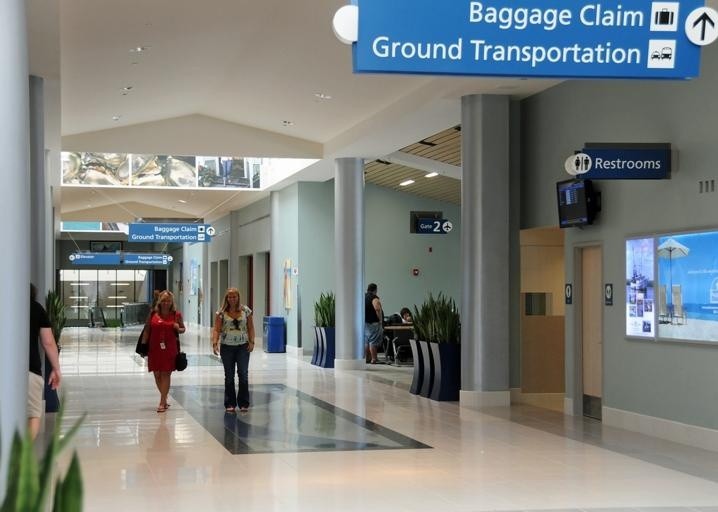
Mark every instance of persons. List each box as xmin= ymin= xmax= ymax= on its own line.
xmin=213 ymin=415 xmax=248 ymax=475
xmin=213 ymin=288 xmax=256 ymax=414
xmin=400 ymin=308 xmax=413 ymax=324
xmin=220 ymin=156 xmax=233 ymax=187
xmin=147 ymin=413 xmax=178 ymax=480
xmin=139 ymin=290 xmax=186 ymax=411
xmin=364 ymin=283 xmax=385 ymax=364
xmin=27 ymin=283 xmax=62 ymax=442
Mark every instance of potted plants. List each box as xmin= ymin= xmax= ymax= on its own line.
xmin=310 ymin=292 xmax=335 ymax=368
xmin=408 ymin=289 xmax=460 ymax=402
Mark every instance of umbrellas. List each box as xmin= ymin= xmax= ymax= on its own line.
xmin=657 ymin=238 xmax=689 ymax=316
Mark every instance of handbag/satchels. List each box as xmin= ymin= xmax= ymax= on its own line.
xmin=176 ymin=348 xmax=190 ymax=373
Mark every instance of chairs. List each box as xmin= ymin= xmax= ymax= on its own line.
xmin=381 ymin=314 xmax=413 ymax=366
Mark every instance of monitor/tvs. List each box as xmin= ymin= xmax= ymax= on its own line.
xmin=556 ymin=178 xmax=599 ymax=228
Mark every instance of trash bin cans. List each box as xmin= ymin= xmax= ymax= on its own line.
xmin=262 ymin=316 xmax=284 ymax=353
